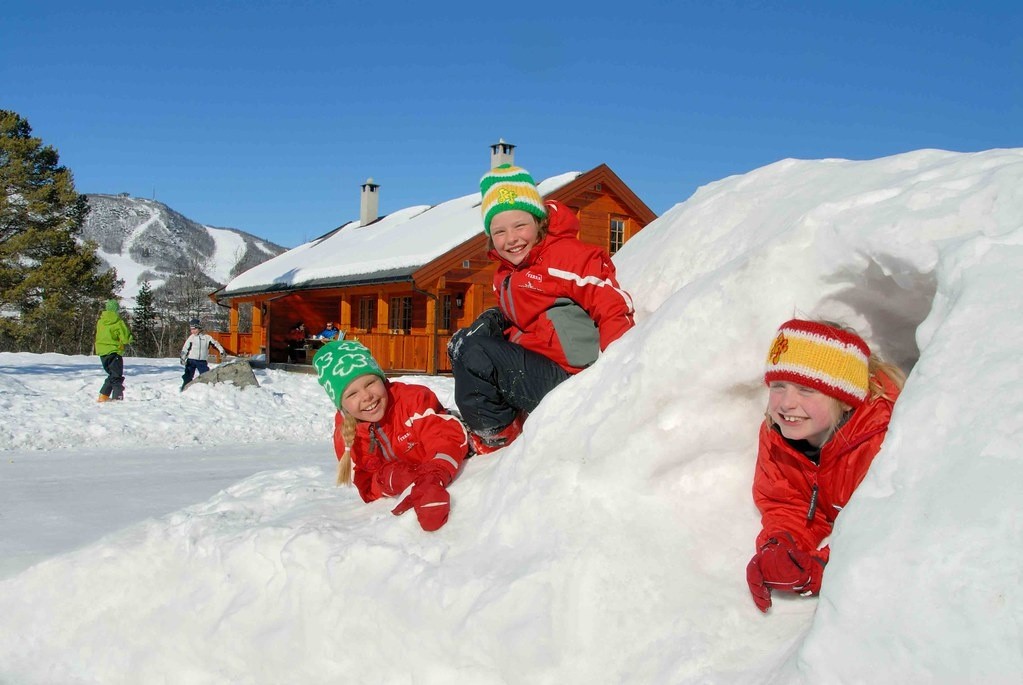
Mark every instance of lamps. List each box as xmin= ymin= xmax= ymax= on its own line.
xmin=456 ymin=293 xmax=463 ymax=308
xmin=262 ymin=304 xmax=267 ymax=315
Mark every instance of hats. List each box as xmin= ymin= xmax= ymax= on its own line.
xmin=190 ymin=319 xmax=204 ymax=328
xmin=312 ymin=339 xmax=388 ymax=411
xmin=479 ymin=163 xmax=548 ymax=238
xmin=106 ymin=299 xmax=119 ymax=312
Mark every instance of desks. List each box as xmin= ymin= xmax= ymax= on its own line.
xmin=306 ymin=339 xmax=330 ymax=345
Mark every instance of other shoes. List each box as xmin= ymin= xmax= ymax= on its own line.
xmin=98 ymin=393 xmax=109 ymax=403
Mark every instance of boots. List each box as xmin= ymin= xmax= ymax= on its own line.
xmin=469 ymin=406 xmax=529 ymax=457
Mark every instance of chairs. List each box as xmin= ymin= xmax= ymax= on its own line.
xmin=288 ymin=345 xmax=310 ymax=363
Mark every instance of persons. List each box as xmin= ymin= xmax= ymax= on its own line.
xmin=179 ymin=319 xmax=225 ymax=391
xmin=745 ymin=319 xmax=908 ymax=612
xmin=316 ymin=322 xmax=339 ymax=339
xmin=95 ymin=300 xmax=133 ymax=403
xmin=447 ymin=163 xmax=635 ymax=456
xmin=312 ymin=341 xmax=469 ymax=532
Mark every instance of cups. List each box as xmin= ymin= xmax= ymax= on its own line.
xmin=313 ymin=335 xmax=316 ymax=340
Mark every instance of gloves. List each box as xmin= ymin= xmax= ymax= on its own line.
xmin=220 ymin=353 xmax=227 ymax=358
xmin=760 ymin=543 xmax=827 ymax=597
xmin=374 ymin=458 xmax=423 ymax=498
xmin=391 ymin=463 xmax=450 ymax=532
xmin=180 ymin=358 xmax=186 ymax=365
xmin=746 ymin=553 xmax=772 ymax=613
